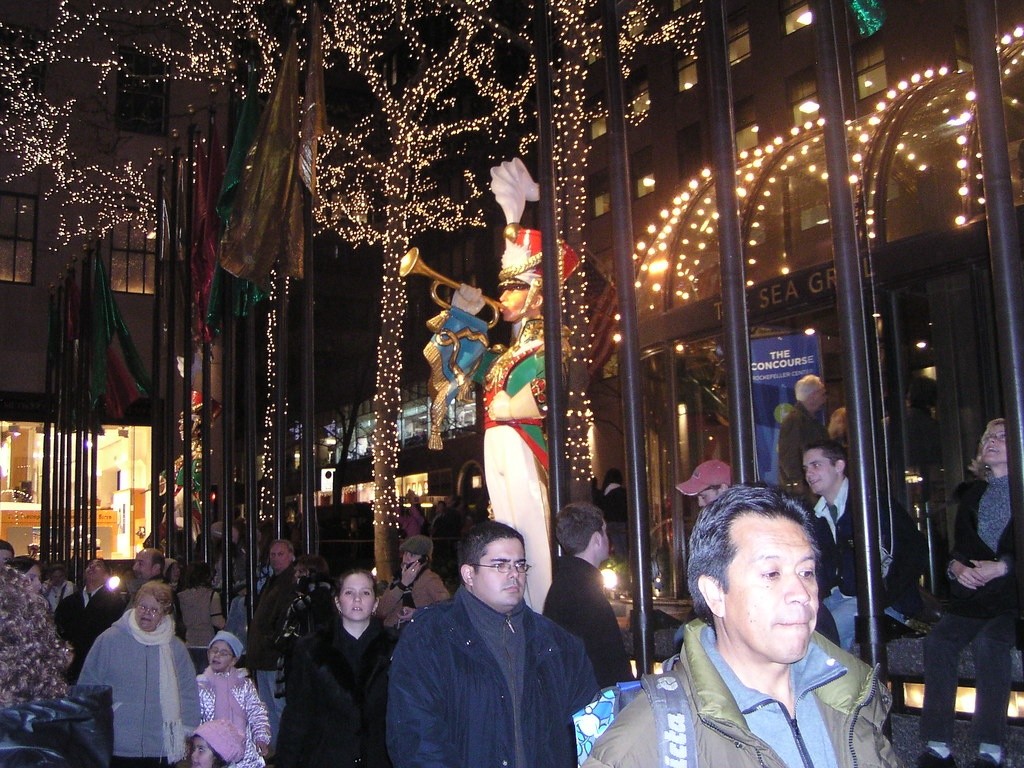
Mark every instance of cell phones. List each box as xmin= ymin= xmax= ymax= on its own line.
xmin=407 ymin=555 xmax=430 ymax=571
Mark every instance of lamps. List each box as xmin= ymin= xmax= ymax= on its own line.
xmin=35 ymin=423 xmax=44 ymax=433
xmin=9 ymin=423 xmax=20 ymax=432
xmin=118 ymin=427 xmax=128 ymax=436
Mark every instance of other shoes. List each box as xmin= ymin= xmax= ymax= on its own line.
xmin=969 ymin=754 xmax=1007 ymax=768
xmin=918 ymin=750 xmax=957 ymax=768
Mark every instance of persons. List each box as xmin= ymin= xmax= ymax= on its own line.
xmin=423 ymin=227 xmax=581 ymax=611
xmin=541 ymin=503 xmax=637 ymax=688
xmin=185 ymin=719 xmax=246 ymax=768
xmin=196 ymin=631 xmax=272 ymax=768
xmin=584 ymin=483 xmax=905 ymax=768
xmin=917 ymin=417 xmax=1024 ymax=768
xmin=0 ymin=563 xmax=114 ymax=768
xmin=76 ymin=581 xmax=202 ymax=768
xmin=386 ymin=520 xmax=599 ymax=768
xmin=801 ymin=442 xmax=923 ymax=652
xmin=0 ymin=517 xmax=343 ymax=750
xmin=777 ymin=374 xmax=945 ymax=527
xmin=379 ymin=534 xmax=450 ymax=629
xmin=274 ymin=568 xmax=401 ymax=768
xmin=158 ymin=392 xmax=223 ymax=537
xmin=589 ymin=467 xmax=628 ymax=563
xmin=675 ymin=459 xmax=731 ymax=507
xmin=320 ymin=493 xmax=473 ymax=586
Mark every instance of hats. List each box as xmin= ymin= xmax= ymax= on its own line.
xmin=193 ymin=719 xmax=246 ymax=763
xmin=675 ymin=459 xmax=731 ymax=495
xmin=400 ymin=535 xmax=433 ymax=559
xmin=209 ymin=630 xmax=244 ymax=659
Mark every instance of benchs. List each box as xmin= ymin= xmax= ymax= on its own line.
xmin=618 ymin=627 xmax=1024 ymax=768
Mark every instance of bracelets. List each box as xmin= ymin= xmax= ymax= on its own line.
xmin=947 ymin=559 xmax=956 ymax=580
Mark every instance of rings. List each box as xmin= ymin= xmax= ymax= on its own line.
xmin=411 ymin=569 xmax=414 ymax=571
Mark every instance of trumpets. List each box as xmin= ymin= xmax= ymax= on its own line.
xmin=398 ymin=248 xmax=504 ymax=330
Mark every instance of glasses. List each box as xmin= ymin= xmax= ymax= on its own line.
xmin=981 ymin=431 xmax=1006 ymax=444
xmin=137 ymin=603 xmax=163 ymax=617
xmin=473 ymin=562 xmax=532 ymax=573
xmin=210 ymin=647 xmax=232 ymax=658
